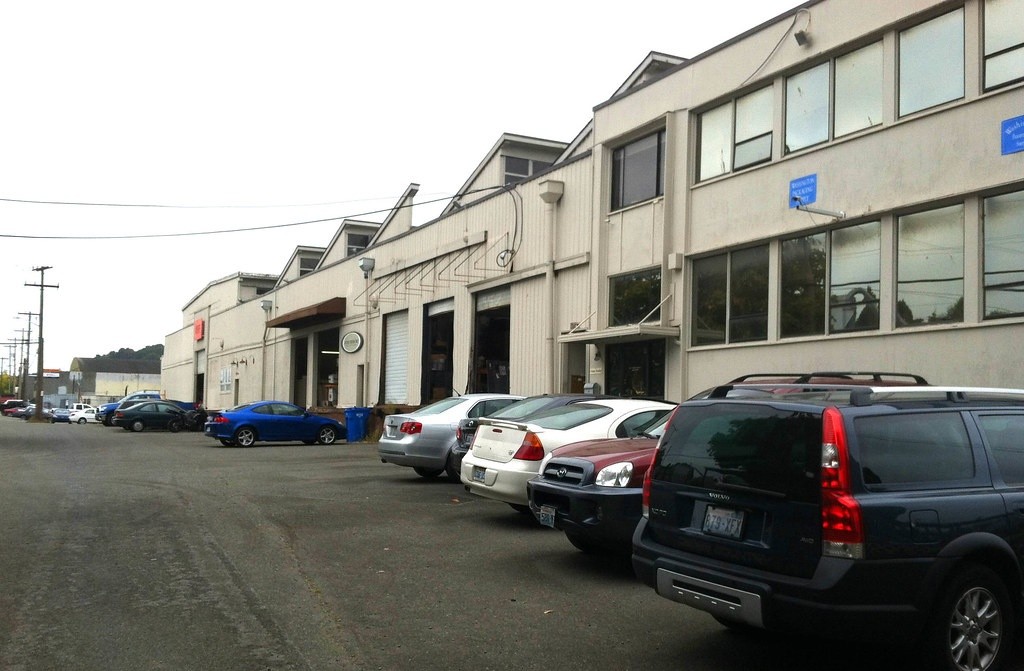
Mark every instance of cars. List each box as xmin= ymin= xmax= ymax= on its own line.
xmin=68 ymin=408 xmax=102 ymax=424
xmin=449 ymin=393 xmax=681 ymax=477
xmin=204 ymin=401 xmax=347 ymax=448
xmin=0 ymin=399 xmax=53 ymax=421
xmin=110 ymin=400 xmax=206 ymax=432
xmin=458 ymin=399 xmax=679 ymax=513
xmin=377 ymin=393 xmax=525 ymax=483
xmin=52 ymin=409 xmax=73 ymax=424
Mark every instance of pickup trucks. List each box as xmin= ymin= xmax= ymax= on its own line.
xmin=51 ymin=403 xmax=93 ymax=413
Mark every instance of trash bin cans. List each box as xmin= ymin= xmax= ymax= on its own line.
xmin=345 ymin=407 xmax=370 ymax=445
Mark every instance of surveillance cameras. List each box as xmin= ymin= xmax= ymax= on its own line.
xmin=793 ymin=195 xmax=802 ymax=201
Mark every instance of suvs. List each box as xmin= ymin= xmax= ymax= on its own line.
xmin=628 ymin=373 xmax=1024 ymax=671
xmin=526 ymin=369 xmax=937 ymax=551
xmin=94 ymin=390 xmax=160 ymax=427
